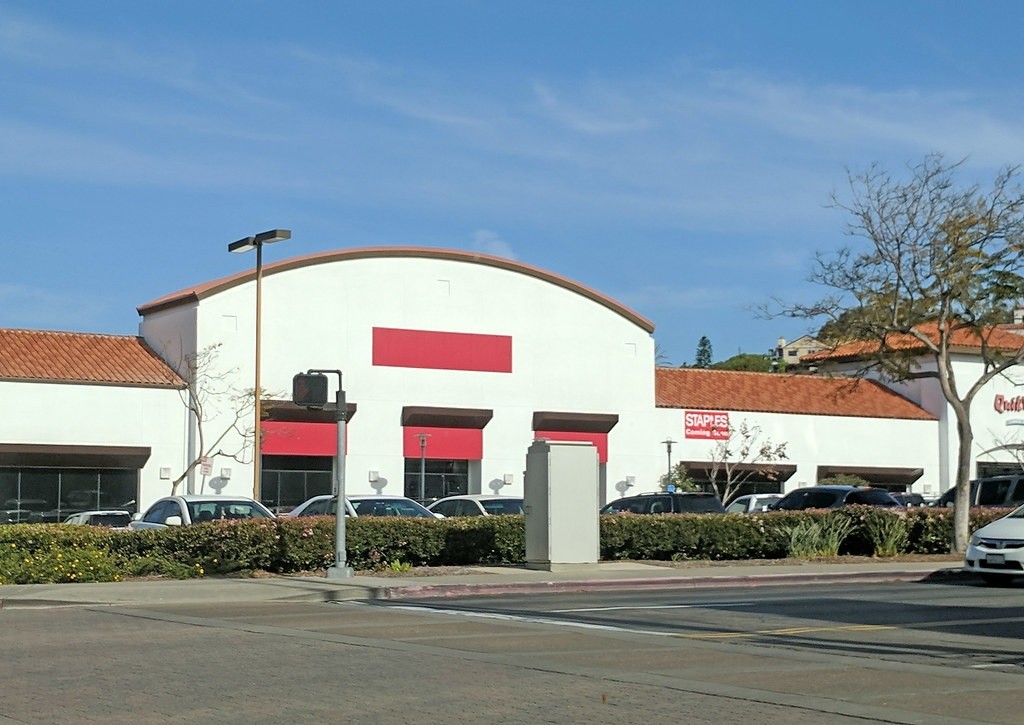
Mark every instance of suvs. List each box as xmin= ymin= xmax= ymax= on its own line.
xmin=600 ymin=491 xmax=729 ymax=514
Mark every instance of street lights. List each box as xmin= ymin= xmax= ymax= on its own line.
xmin=660 ymin=437 xmax=678 ymax=492
xmin=228 ymin=228 xmax=292 ymax=502
xmin=414 ymin=433 xmax=433 ymax=507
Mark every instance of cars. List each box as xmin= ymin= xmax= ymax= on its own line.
xmin=932 ymin=475 xmax=1024 ymax=508
xmin=275 ymin=494 xmax=448 ymax=520
xmin=128 ymin=494 xmax=277 ymax=530
xmin=414 ymin=494 xmax=524 ymax=518
xmin=964 ymin=504 xmax=1024 ymax=583
xmin=889 ymin=492 xmax=927 ymax=508
xmin=62 ymin=510 xmax=133 ymax=532
xmin=767 ymin=486 xmax=902 ymax=511
xmin=725 ymin=493 xmax=786 ymax=514
